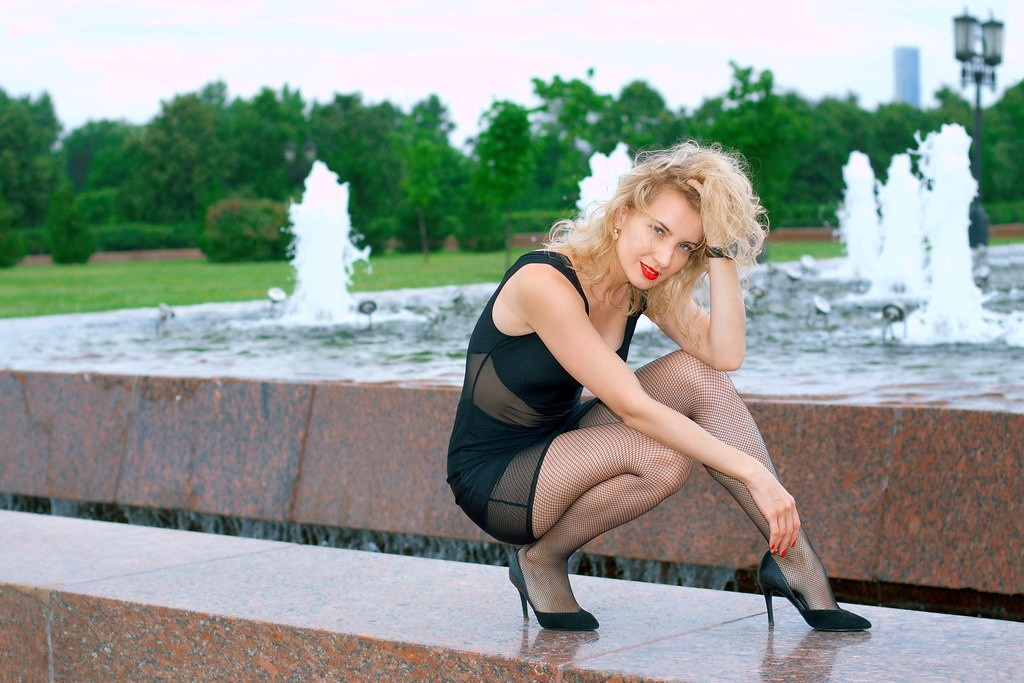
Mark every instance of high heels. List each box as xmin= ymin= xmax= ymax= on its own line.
xmin=757 ymin=549 xmax=872 ymax=631
xmin=509 ymin=545 xmax=600 ymax=631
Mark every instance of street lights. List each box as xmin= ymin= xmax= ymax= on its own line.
xmin=954 ymin=3 xmax=1003 ymax=249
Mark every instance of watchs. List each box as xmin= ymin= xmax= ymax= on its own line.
xmin=702 ymin=243 xmax=739 ymax=260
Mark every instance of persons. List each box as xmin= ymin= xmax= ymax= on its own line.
xmin=445 ymin=136 xmax=872 ymax=631
